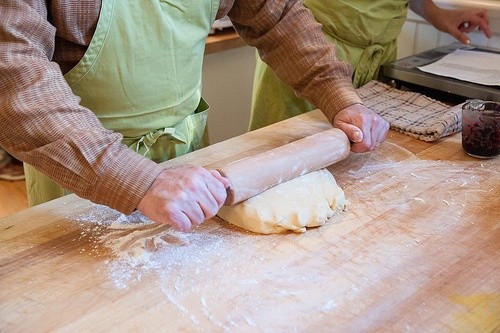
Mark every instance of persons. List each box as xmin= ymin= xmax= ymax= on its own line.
xmin=0 ymin=0 xmax=390 ymax=231
xmin=248 ymin=0 xmax=493 ymax=132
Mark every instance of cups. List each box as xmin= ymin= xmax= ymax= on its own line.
xmin=460 ymin=100 xmax=500 ymax=159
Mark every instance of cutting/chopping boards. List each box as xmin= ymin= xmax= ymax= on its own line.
xmin=0 ymin=107 xmax=500 ymax=333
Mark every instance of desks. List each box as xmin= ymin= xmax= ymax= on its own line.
xmin=202 ymin=33 xmax=257 ymax=145
xmin=0 ymin=108 xmax=500 ymax=333
